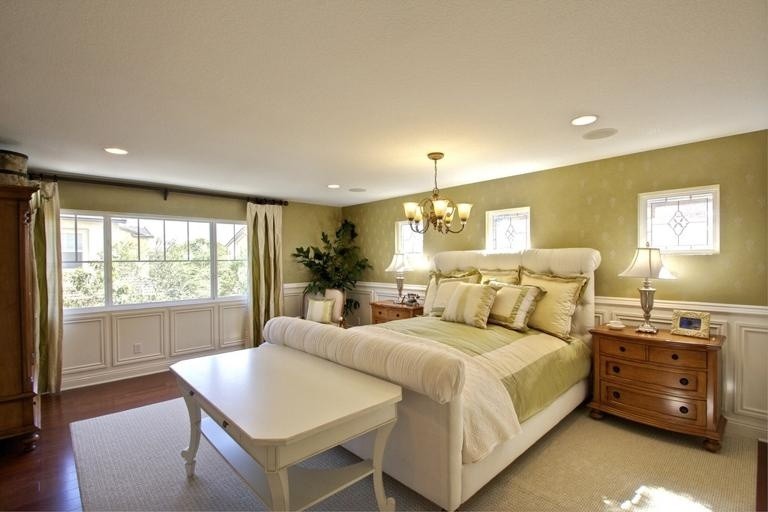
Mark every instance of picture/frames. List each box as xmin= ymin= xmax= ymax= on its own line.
xmin=671 ymin=310 xmax=711 ymax=339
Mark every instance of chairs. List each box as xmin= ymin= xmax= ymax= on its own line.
xmin=295 ymin=286 xmax=347 ymax=329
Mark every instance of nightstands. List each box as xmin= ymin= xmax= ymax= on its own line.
xmin=369 ymin=300 xmax=423 ymax=324
xmin=589 ymin=323 xmax=727 ymax=451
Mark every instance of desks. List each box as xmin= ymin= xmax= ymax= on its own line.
xmin=169 ymin=344 xmax=402 ymax=512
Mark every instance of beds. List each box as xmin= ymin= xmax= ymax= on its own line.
xmin=262 ymin=248 xmax=602 ymax=512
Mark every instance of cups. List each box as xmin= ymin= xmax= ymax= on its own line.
xmin=610 ymin=321 xmax=622 ymax=326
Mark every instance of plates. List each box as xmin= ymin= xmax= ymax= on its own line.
xmin=606 ymin=323 xmax=626 ymax=330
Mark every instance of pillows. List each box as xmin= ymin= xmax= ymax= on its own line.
xmin=423 ymin=265 xmax=589 ymax=343
xmin=306 ymin=297 xmax=335 ymax=325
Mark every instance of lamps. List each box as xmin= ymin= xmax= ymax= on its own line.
xmin=617 ymin=241 xmax=677 ymax=334
xmin=384 ymin=253 xmax=414 ymax=304
xmin=403 ymin=152 xmax=474 ymax=235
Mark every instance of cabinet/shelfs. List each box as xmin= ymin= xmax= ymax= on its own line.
xmin=0 ymin=184 xmax=42 ymax=452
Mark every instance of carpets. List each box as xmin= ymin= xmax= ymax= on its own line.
xmin=68 ymin=397 xmax=768 ymax=512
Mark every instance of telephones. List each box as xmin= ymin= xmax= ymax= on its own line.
xmin=401 ymin=293 xmax=420 ymax=306
